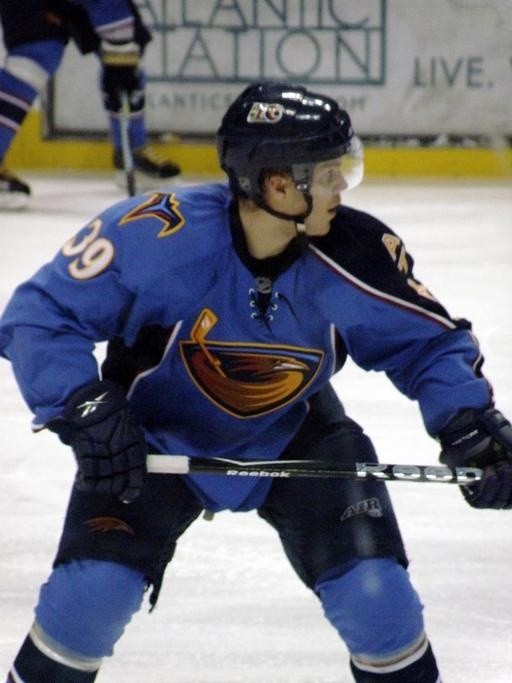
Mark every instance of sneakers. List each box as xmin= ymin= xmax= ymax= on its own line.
xmin=112 ymin=143 xmax=181 ymax=178
xmin=0 ymin=171 xmax=30 ymax=195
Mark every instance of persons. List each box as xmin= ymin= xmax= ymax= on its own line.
xmin=1 ymin=84 xmax=510 ymax=681
xmin=1 ymin=0 xmax=181 ymax=195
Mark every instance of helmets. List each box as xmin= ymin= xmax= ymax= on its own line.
xmin=214 ymin=79 xmax=365 ymax=201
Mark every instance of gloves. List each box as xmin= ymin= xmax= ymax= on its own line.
xmin=437 ymin=405 xmax=511 ymax=510
xmin=45 ymin=382 xmax=147 ymax=506
